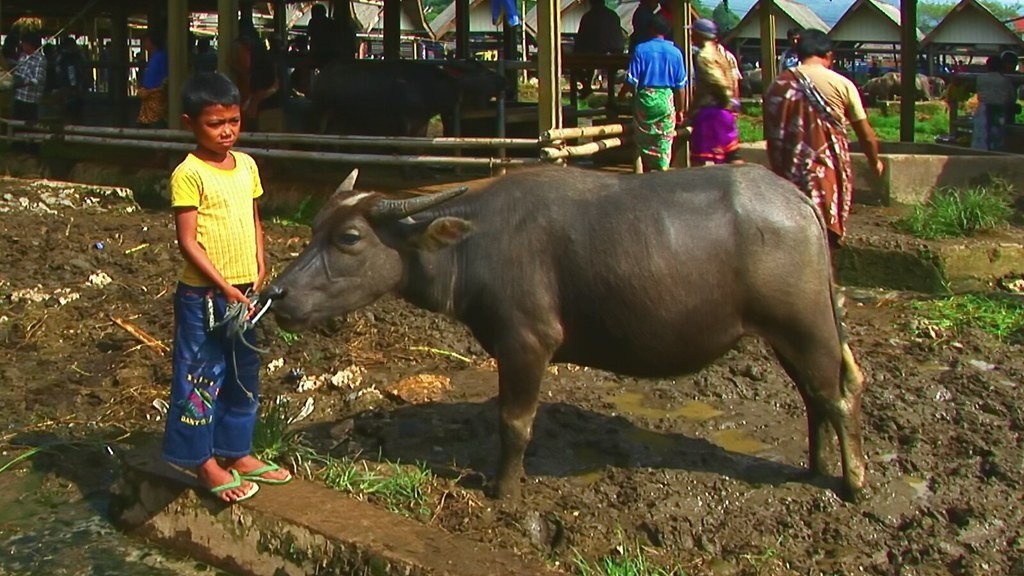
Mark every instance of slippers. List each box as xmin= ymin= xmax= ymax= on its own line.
xmin=240 ymin=459 xmax=292 ymax=484
xmin=209 ymin=468 xmax=260 ymax=503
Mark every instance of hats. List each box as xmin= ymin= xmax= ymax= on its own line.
xmin=690 ymin=18 xmax=718 ymax=39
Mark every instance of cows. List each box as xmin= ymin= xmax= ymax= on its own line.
xmin=259 ymin=164 xmax=868 ymax=499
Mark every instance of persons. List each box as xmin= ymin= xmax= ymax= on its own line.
xmin=2 ymin=26 xmax=113 ymax=161
xmin=866 ymin=62 xmax=880 ymax=82
xmin=574 ymin=0 xmax=674 ymax=115
xmin=944 ymin=60 xmax=968 ymax=78
xmin=161 ymin=71 xmax=292 ymax=503
xmin=779 ymin=28 xmax=803 ymax=71
xmin=618 ymin=14 xmax=687 ymax=174
xmin=976 ymin=51 xmax=1024 ymax=126
xmin=761 ymin=29 xmax=883 ymax=289
xmin=690 ymin=19 xmax=743 ymax=168
xmin=129 ymin=0 xmax=355 ymax=181
